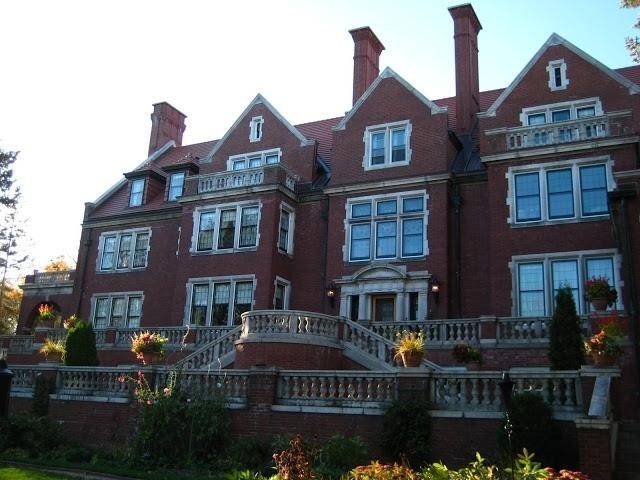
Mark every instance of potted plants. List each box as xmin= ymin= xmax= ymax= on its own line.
xmin=453 ymin=343 xmax=486 ymax=371
xmin=40 ymin=338 xmax=66 ymax=362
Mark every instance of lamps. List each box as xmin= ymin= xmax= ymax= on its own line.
xmin=326 ymin=284 xmax=336 ymax=305
xmin=431 ymin=274 xmax=440 ymax=304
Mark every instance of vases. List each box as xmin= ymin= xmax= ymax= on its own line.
xmin=592 ymin=349 xmax=616 ymax=366
xmin=592 ymin=298 xmax=609 ymax=310
xmin=43 ymin=318 xmax=54 ymax=328
xmin=139 ymin=352 xmax=153 ymax=363
xmin=401 ymin=351 xmax=422 ymax=368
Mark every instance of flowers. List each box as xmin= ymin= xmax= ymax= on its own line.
xmin=130 ymin=331 xmax=168 ymax=358
xmin=393 ymin=329 xmax=431 ymax=360
xmin=583 ymin=310 xmax=629 ymax=357
xmin=38 ymin=303 xmax=58 ymax=319
xmin=582 ymin=276 xmax=619 ymax=308
